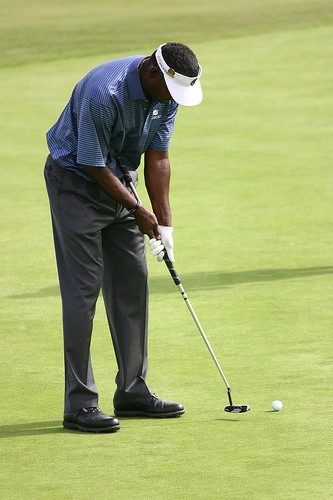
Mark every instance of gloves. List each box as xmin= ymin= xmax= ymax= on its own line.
xmin=148 ymin=224 xmax=175 ymax=264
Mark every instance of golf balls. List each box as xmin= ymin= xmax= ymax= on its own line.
xmin=271 ymin=400 xmax=283 ymax=411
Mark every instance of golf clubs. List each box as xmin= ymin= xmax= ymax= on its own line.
xmin=119 ymin=158 xmax=251 ymax=414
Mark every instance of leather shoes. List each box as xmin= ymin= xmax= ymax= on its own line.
xmin=113 ymin=392 xmax=185 ymax=417
xmin=64 ymin=407 xmax=120 ymax=432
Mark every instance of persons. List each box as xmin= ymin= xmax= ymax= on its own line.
xmin=43 ymin=42 xmax=200 ymax=433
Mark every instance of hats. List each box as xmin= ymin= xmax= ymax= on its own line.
xmin=155 ymin=43 xmax=203 ymax=106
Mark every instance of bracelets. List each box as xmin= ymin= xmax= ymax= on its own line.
xmin=129 ymin=202 xmax=140 ymax=212
